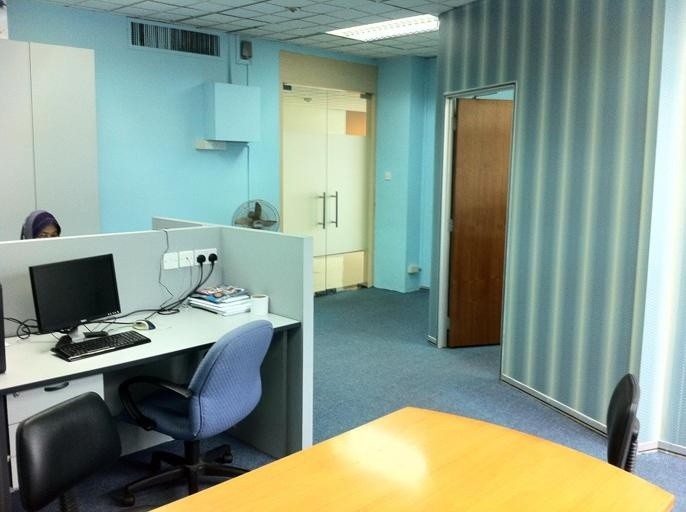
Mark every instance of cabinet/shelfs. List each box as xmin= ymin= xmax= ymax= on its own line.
xmin=0 ymin=38 xmax=100 ymax=245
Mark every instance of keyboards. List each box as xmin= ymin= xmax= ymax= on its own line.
xmin=51 ymin=328 xmax=151 ymax=363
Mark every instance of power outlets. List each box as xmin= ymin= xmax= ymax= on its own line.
xmin=195 ymin=248 xmax=218 ymax=266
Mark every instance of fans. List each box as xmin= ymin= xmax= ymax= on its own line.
xmin=231 ymin=199 xmax=280 ymax=232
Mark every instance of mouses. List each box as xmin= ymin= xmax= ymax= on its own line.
xmin=134 ymin=317 xmax=155 ymax=331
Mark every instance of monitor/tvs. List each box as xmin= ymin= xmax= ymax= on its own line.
xmin=28 ymin=253 xmax=122 ymax=346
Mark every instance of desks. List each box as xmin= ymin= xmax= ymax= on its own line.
xmin=148 ymin=407 xmax=675 ymax=512
xmin=0 ymin=304 xmax=301 ymax=512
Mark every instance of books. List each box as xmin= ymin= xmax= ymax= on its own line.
xmin=186 ymin=282 xmax=253 ymax=317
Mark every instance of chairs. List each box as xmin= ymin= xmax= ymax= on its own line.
xmin=16 ymin=392 xmax=121 ymax=512
xmin=606 ymin=373 xmax=640 ymax=475
xmin=109 ymin=319 xmax=275 ymax=507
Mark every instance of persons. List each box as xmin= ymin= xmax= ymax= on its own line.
xmin=19 ymin=209 xmax=62 ymax=240
